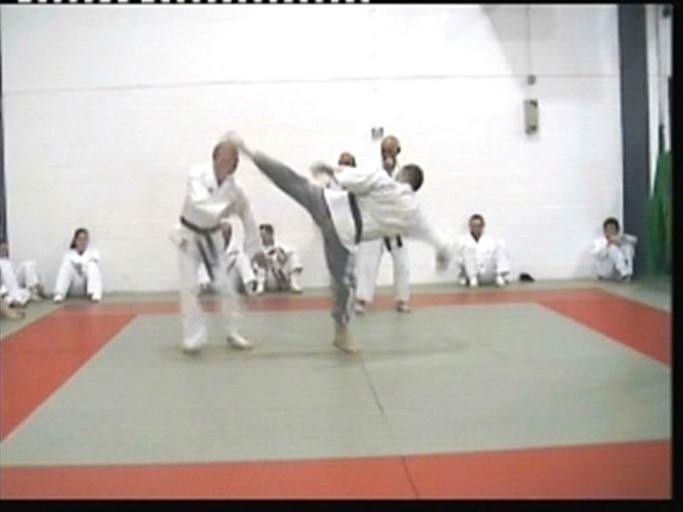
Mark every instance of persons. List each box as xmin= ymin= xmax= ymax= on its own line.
xmin=0 ymin=240 xmax=42 ymax=306
xmin=173 ymin=139 xmax=269 ymax=353
xmin=593 ymin=217 xmax=638 ymax=284
xmin=52 ymin=228 xmax=103 ymax=303
xmin=456 ymin=214 xmax=510 ymax=287
xmin=0 ymin=296 xmax=26 ymax=320
